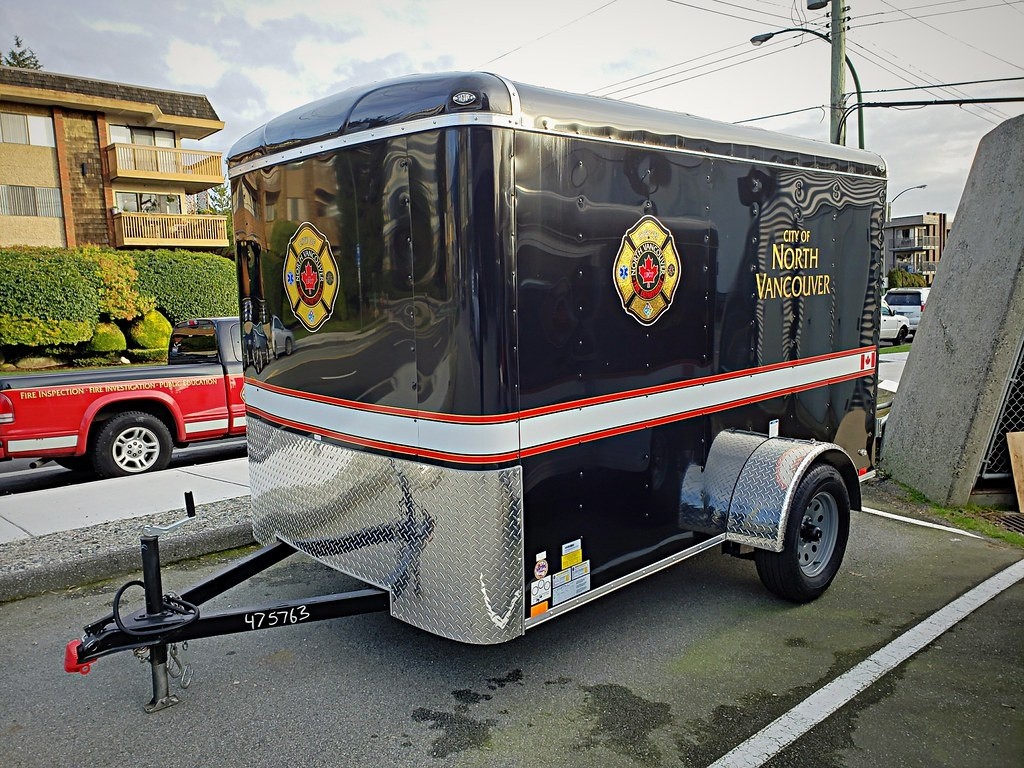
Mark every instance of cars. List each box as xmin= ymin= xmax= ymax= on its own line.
xmin=247 ymin=321 xmax=273 ymax=376
xmin=880 ymin=298 xmax=911 ymax=345
xmin=881 ymin=286 xmax=933 ymax=337
xmin=270 ymin=314 xmax=296 ymax=361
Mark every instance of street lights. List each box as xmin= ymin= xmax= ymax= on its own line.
xmin=887 ymin=182 xmax=929 ymax=221
xmin=749 ymin=27 xmax=865 ymax=152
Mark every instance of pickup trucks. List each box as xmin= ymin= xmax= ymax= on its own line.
xmin=0 ymin=316 xmax=249 ymax=482
